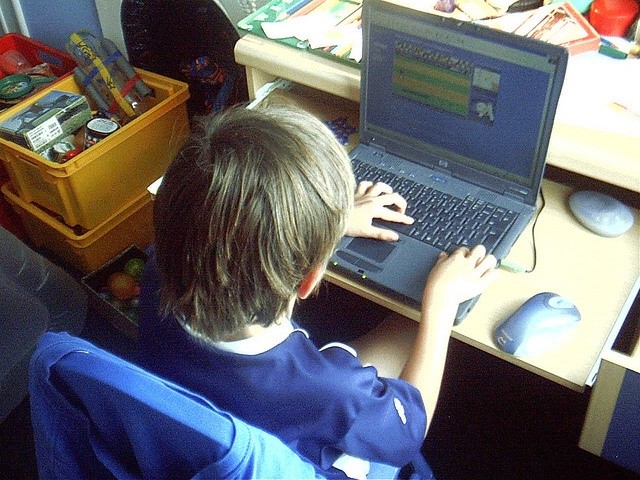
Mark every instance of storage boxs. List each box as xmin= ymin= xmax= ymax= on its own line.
xmin=82 ymin=244 xmax=148 ymax=330
xmin=1 ymin=66 xmax=190 ymax=230
xmin=0 ymin=31 xmax=79 ymax=110
xmin=0 ymin=181 xmax=169 ymax=272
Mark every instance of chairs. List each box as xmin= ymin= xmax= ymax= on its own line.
xmin=28 ymin=329 xmax=326 ymax=480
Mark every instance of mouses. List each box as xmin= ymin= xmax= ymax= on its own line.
xmin=493 ymin=291 xmax=584 ymax=356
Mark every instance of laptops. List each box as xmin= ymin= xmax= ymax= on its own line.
xmin=312 ymin=0 xmax=569 ymax=325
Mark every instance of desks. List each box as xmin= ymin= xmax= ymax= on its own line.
xmin=145 ymin=0 xmax=640 ymax=477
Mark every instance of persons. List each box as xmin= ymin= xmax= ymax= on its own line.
xmin=131 ymin=95 xmax=498 ymax=480
